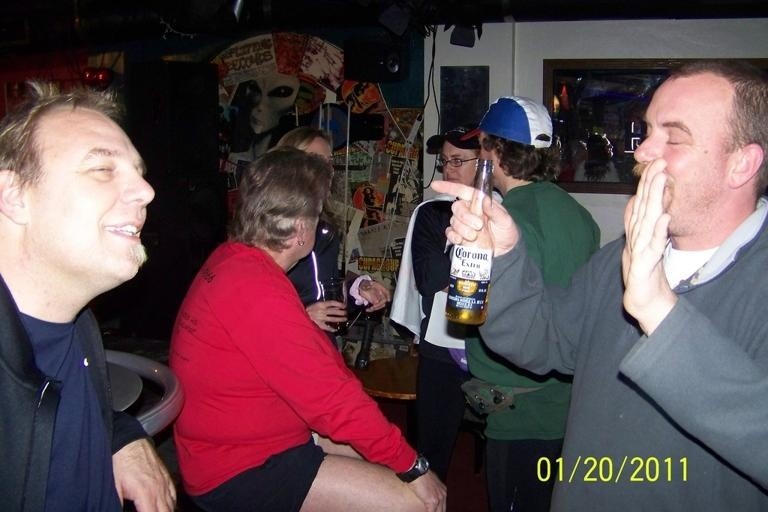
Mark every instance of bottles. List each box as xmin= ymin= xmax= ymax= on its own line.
xmin=444 ymin=158 xmax=494 ymax=326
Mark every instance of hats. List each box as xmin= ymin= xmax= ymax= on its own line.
xmin=460 ymin=96 xmax=554 ymax=149
xmin=425 ymin=124 xmax=483 ymax=155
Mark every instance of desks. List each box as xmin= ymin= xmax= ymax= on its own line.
xmin=347 ymin=355 xmax=418 ymax=401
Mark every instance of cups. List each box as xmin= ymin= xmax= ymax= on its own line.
xmin=323 ymin=277 xmax=348 ymax=335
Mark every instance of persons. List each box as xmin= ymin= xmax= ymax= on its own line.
xmin=460 ymin=94 xmax=602 ymax=512
xmin=428 ymin=58 xmax=768 ymax=512
xmin=389 ymin=124 xmax=505 ymax=482
xmin=272 ymin=126 xmax=391 ymax=354
xmin=169 ymin=147 xmax=448 ymax=511
xmin=0 ymin=76 xmax=178 ymax=511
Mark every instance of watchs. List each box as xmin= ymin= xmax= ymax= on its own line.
xmin=396 ymin=450 xmax=430 ymax=482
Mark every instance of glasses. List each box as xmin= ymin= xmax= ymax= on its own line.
xmin=434 ymin=156 xmax=479 ymax=168
